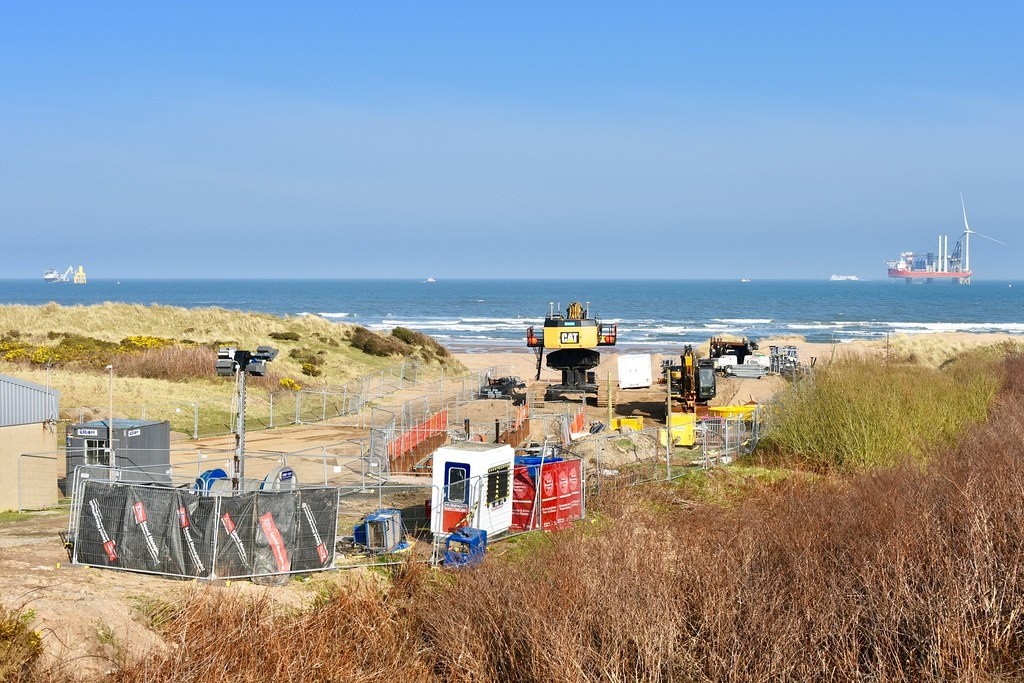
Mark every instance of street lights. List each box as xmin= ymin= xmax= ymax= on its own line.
xmin=105 ymin=363 xmax=114 ymax=483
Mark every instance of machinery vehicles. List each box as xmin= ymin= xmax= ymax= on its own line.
xmin=656 ymin=344 xmax=717 ymax=421
xmin=525 ymin=302 xmax=620 ymax=409
